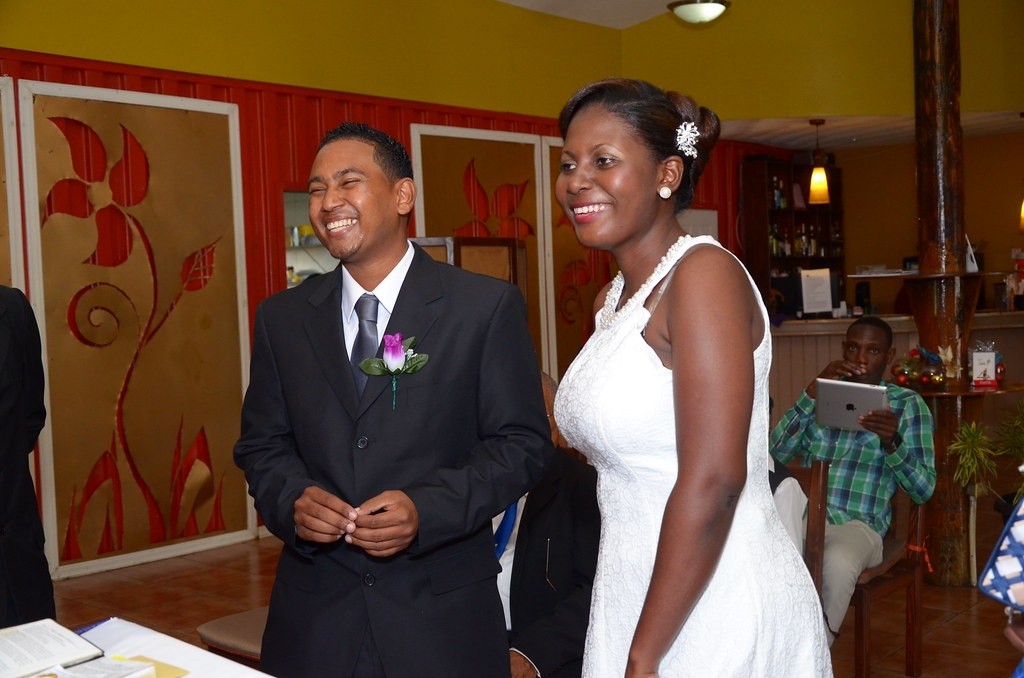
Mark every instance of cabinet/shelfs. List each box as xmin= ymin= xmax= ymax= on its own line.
xmin=847 ymin=265 xmax=1019 ymax=588
xmin=744 ymin=156 xmax=845 ymax=307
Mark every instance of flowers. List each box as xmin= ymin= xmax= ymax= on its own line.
xmin=360 ymin=332 xmax=426 ymax=408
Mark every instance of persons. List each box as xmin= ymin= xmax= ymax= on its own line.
xmin=0 ymin=285 xmax=55 ymax=629
xmin=490 ymin=446 xmax=601 ymax=678
xmin=552 ymin=77 xmax=834 ymax=678
xmin=768 ymin=318 xmax=937 ymax=649
xmin=232 ymin=120 xmax=551 ymax=678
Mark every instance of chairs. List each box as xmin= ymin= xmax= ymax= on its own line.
xmin=854 ymin=487 xmax=934 ymax=678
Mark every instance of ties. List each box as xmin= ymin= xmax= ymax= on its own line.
xmin=492 ymin=501 xmax=517 ymax=560
xmin=350 ymin=293 xmax=380 ymax=402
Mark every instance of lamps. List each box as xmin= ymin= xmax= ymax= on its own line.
xmin=667 ymin=0 xmax=730 ymax=24
xmin=809 ymin=118 xmax=830 ymax=204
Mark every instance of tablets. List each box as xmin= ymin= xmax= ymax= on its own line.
xmin=816 ymin=379 xmax=887 ymax=433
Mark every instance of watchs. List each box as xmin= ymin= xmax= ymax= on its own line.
xmin=879 ymin=431 xmax=903 ymax=452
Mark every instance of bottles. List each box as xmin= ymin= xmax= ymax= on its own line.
xmin=768 ymin=223 xmax=828 ymax=256
xmin=772 ymin=175 xmax=785 ymax=208
